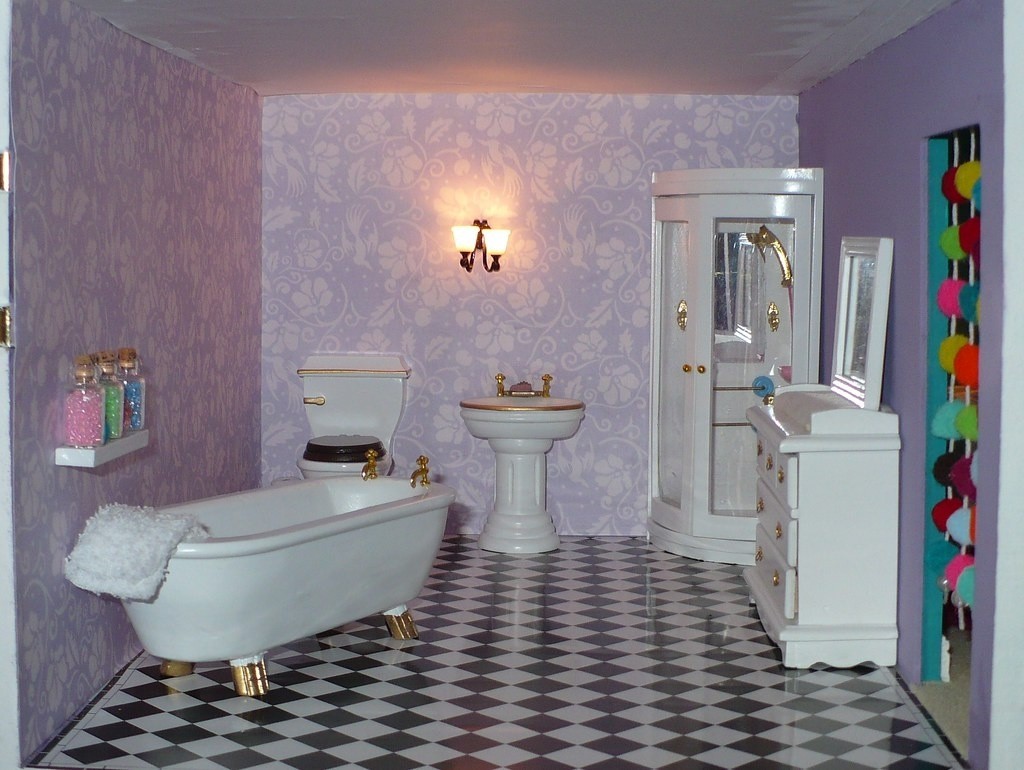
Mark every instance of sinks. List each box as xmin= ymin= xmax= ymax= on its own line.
xmin=459 ymin=388 xmax=588 ymax=442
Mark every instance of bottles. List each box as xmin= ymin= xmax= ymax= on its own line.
xmin=97 ymin=351 xmax=124 ymax=438
xmin=65 ymin=356 xmax=105 ymax=448
xmin=118 ymin=349 xmax=146 ymax=431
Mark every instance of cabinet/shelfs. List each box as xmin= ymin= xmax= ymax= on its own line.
xmin=742 ymin=384 xmax=899 ymax=670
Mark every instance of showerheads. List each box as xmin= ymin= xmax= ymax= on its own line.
xmin=744 ymin=223 xmax=793 ymax=289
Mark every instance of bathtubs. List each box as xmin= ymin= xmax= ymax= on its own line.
xmin=100 ymin=475 xmax=460 ymax=696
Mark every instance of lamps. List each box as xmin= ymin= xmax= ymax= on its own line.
xmin=451 ymin=220 xmax=511 ymax=273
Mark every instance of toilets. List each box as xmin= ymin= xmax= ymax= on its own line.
xmin=297 ymin=348 xmax=413 ymax=480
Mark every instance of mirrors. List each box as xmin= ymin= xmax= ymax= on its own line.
xmin=829 ymin=236 xmax=895 ymax=410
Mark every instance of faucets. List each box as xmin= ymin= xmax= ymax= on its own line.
xmin=360 ymin=447 xmax=379 ymax=482
xmin=407 ymin=454 xmax=433 ymax=488
xmin=495 ymin=372 xmax=554 ymax=398
